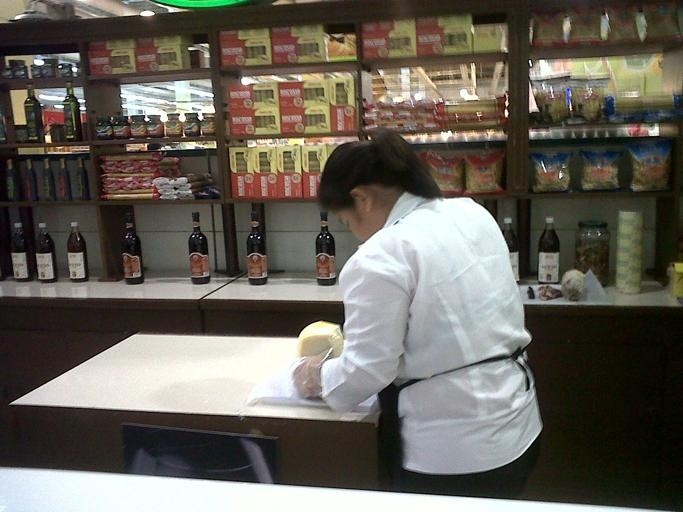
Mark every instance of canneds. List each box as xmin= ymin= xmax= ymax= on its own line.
xmin=95 ymin=111 xmax=216 ymax=140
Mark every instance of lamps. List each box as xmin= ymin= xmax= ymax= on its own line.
xmin=139 ymin=2 xmax=155 ymax=17
xmin=32 ymin=53 xmax=46 ymax=66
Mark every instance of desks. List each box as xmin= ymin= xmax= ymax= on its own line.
xmin=5 ymin=332 xmax=381 ymax=484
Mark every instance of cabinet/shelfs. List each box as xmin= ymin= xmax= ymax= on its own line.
xmin=523 ymin=303 xmax=683 ymax=461
xmin=0 ymin=1 xmax=682 ymax=275
xmin=1 ymin=294 xmax=201 ymax=377
xmin=200 ymin=298 xmax=344 ymax=337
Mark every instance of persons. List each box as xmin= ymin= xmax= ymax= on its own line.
xmin=291 ymin=128 xmax=545 ymax=499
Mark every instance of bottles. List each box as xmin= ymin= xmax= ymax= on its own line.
xmin=0 ymin=102 xmax=7 ymax=143
xmin=121 ymin=212 xmax=144 ymax=284
xmin=23 ymin=159 xmax=38 ymax=201
xmin=246 ymin=211 xmax=269 ymax=285
xmin=503 ymin=217 xmax=520 ymax=286
xmin=59 ymin=158 xmax=72 ymax=201
xmin=315 ymin=210 xmax=337 ymax=285
xmin=64 ymin=82 xmax=83 ymax=140
xmin=537 ymin=217 xmax=561 ymax=284
xmin=24 ymin=82 xmax=44 ymax=141
xmin=4 ymin=158 xmax=20 ymax=201
xmin=10 ymin=221 xmax=34 ymax=281
xmin=42 ymin=158 xmax=56 ymax=201
xmin=77 ymin=157 xmax=90 ymax=200
xmin=67 ymin=222 xmax=89 ymax=282
xmin=188 ymin=211 xmax=211 ymax=284
xmin=35 ymin=222 xmax=58 ymax=282
